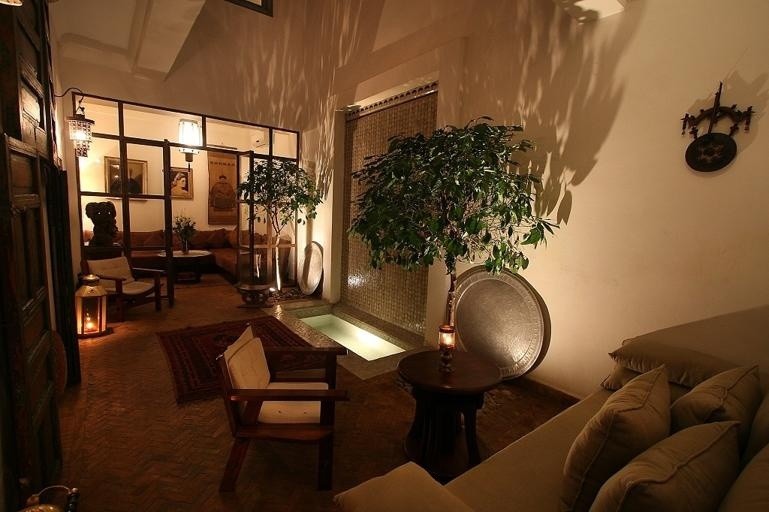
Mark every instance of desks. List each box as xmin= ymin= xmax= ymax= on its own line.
xmin=397 ymin=348 xmax=485 ymax=470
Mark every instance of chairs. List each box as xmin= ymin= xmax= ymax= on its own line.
xmin=214 ymin=326 xmax=338 ymax=495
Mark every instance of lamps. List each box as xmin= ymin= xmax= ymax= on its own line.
xmin=176 ymin=116 xmax=203 ymax=177
xmin=53 ymin=85 xmax=94 ymax=160
xmin=268 ymin=288 xmax=287 ymax=312
xmin=436 ymin=322 xmax=456 ymax=376
xmin=75 ymin=272 xmax=113 ymax=336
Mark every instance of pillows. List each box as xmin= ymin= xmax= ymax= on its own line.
xmin=590 ymin=420 xmax=741 ymax=512
xmin=560 ymin=365 xmax=671 ymax=512
xmin=669 ymin=362 xmax=761 ymax=427
xmin=746 ymin=393 xmax=769 ymax=458
xmin=609 ymin=304 xmax=769 ymax=387
xmin=600 ymin=368 xmax=642 ymax=392
xmin=718 ymin=435 xmax=765 ymax=512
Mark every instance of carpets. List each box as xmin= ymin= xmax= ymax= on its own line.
xmin=154 ymin=317 xmax=341 ymax=407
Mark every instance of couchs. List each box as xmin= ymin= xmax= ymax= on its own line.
xmin=332 ymin=303 xmax=768 ymax=510
